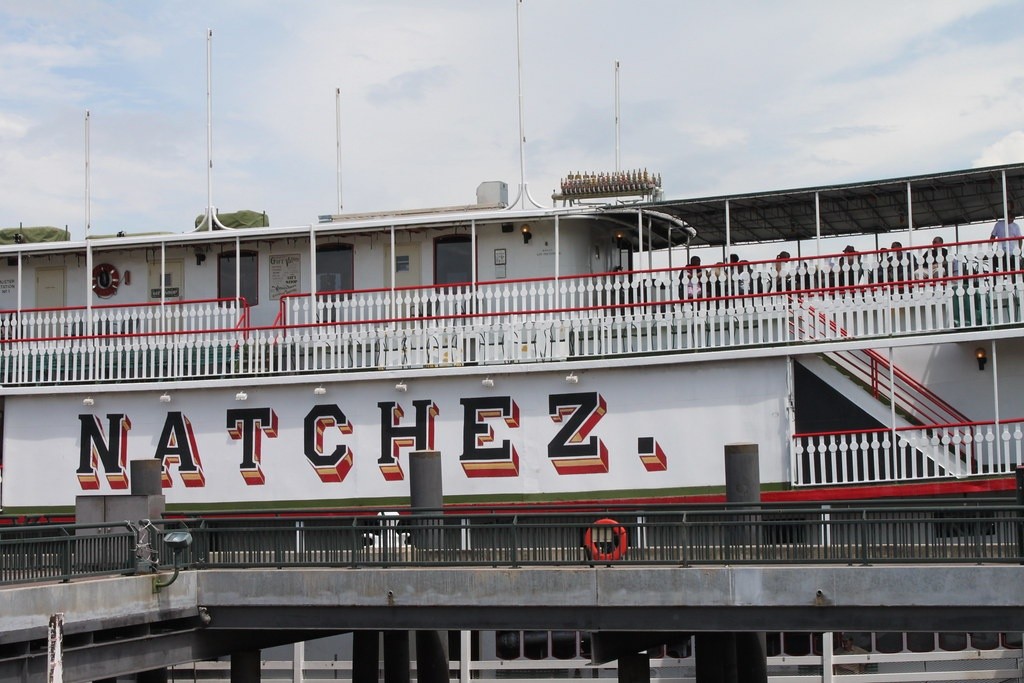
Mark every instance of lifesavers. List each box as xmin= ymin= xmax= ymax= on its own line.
xmin=585 ymin=518 xmax=627 ymax=562
xmin=92 ymin=263 xmax=119 ymax=296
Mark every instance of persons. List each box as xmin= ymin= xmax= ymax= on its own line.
xmin=678 ymin=234 xmax=950 ymax=311
xmin=986 ymin=210 xmax=1023 ymax=288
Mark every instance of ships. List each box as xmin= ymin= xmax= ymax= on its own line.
xmin=0 ymin=3 xmax=1024 ymax=683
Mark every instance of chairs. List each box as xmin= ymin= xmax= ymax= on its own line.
xmin=0 ymin=315 xmax=140 ymax=350
xmin=409 ymin=296 xmax=440 ymax=331
xmin=451 ymin=295 xmax=480 ymax=328
xmin=313 ymin=302 xmax=339 ymax=341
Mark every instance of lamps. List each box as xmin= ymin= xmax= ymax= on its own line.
xmin=151 ymin=531 xmax=194 ymax=594
xmin=520 ymin=223 xmax=532 ymax=244
xmin=193 ymin=246 xmax=207 ymax=266
xmin=974 ymin=346 xmax=989 ymax=371
xmin=615 ymin=229 xmax=626 ymax=249
